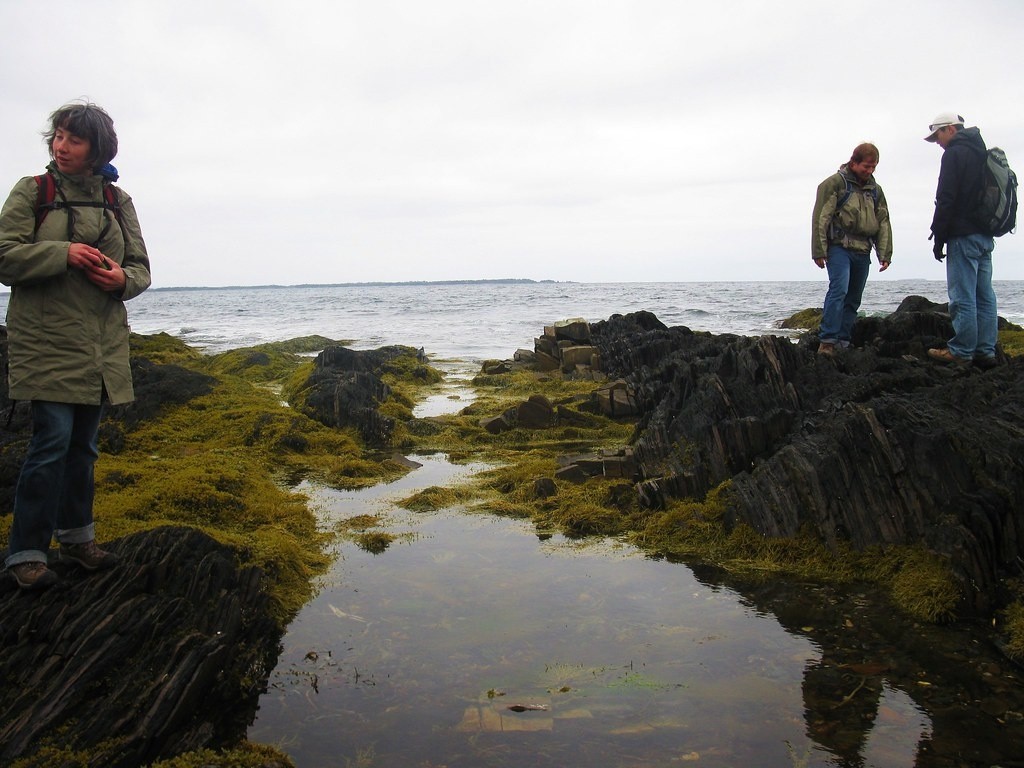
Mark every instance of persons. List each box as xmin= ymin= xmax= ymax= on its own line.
xmin=924 ymin=113 xmax=997 ymax=361
xmin=811 ymin=143 xmax=893 ymax=355
xmin=1 ymin=106 xmax=151 ymax=592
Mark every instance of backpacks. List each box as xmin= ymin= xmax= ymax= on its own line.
xmin=951 ymin=139 xmax=1018 ymax=237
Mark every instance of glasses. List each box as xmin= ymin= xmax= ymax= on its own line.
xmin=929 ymin=123 xmax=952 ymax=132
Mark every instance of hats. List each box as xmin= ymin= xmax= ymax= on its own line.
xmin=924 ymin=112 xmax=965 ymax=142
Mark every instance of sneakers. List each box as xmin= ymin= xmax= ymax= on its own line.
xmin=977 ymin=354 xmax=997 ymax=368
xmin=58 ymin=538 xmax=111 ymax=569
xmin=11 ymin=561 xmax=50 ymax=587
xmin=817 ymin=342 xmax=834 ymax=357
xmin=928 ymin=348 xmax=973 ymax=368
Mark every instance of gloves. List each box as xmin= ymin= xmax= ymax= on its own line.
xmin=933 ymin=238 xmax=946 ymax=262
